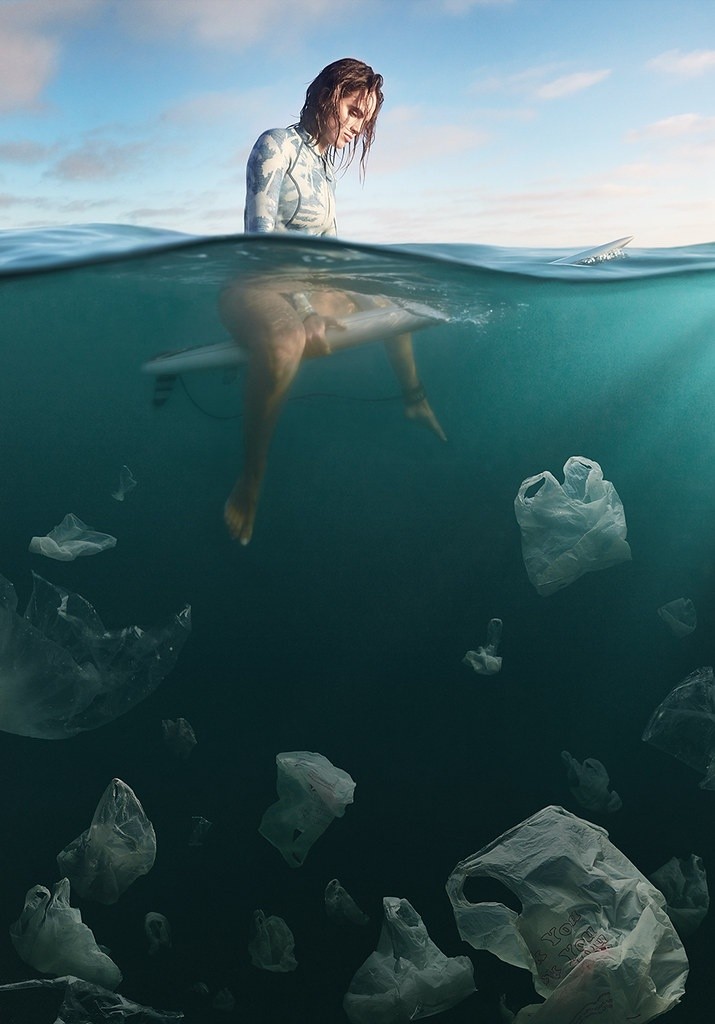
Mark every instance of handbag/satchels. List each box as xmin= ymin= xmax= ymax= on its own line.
xmin=55 ymin=778 xmax=156 ymax=905
xmin=517 ymin=456 xmax=631 ymax=598
xmin=447 ymin=804 xmax=690 ymax=1024
xmin=257 ymin=750 xmax=356 ymax=869
xmin=8 ymin=876 xmax=122 ymax=991
xmin=342 ymin=896 xmax=478 ymax=1024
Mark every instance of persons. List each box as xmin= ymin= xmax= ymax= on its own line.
xmin=244 ymin=58 xmax=383 ymax=235
